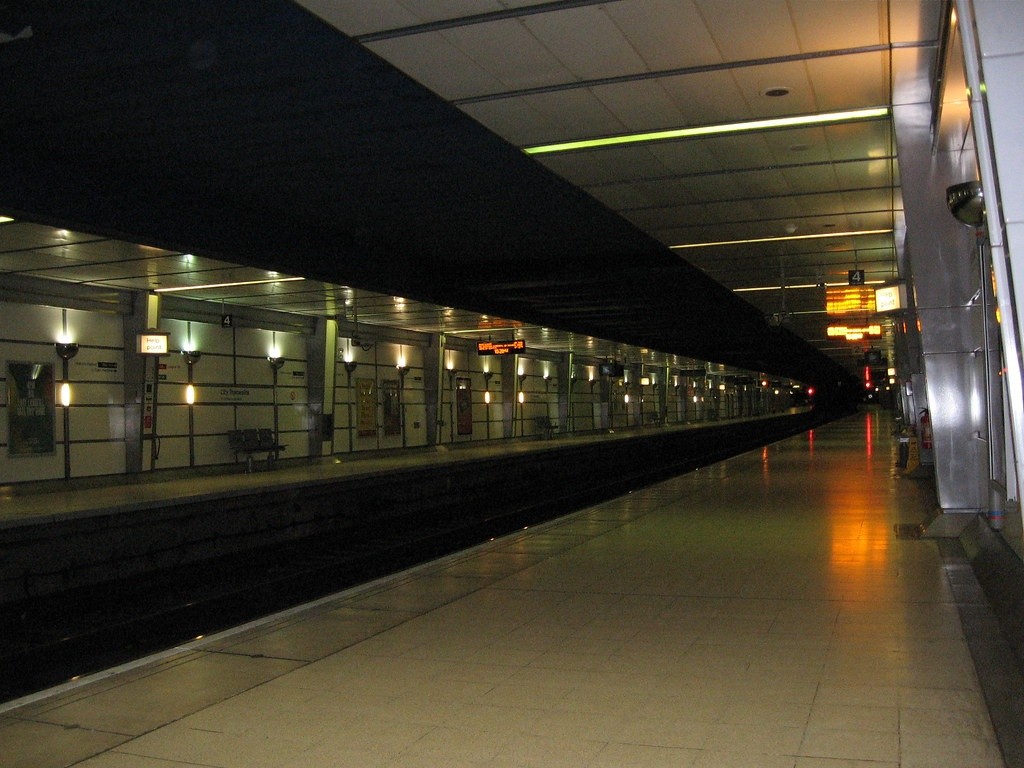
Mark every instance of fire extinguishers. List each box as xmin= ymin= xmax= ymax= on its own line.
xmin=918 ymin=407 xmax=932 ymax=449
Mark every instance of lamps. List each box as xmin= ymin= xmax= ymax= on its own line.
xmin=344 ymin=361 xmax=357 ymax=372
xmin=181 ymin=351 xmax=201 ymax=365
xmin=56 ymin=343 xmax=79 ymax=359
xmin=269 ymin=357 xmax=284 ymax=369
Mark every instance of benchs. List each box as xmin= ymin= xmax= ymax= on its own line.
xmin=229 ymin=428 xmax=289 ymax=473
xmin=535 ymin=416 xmax=558 ymax=440
